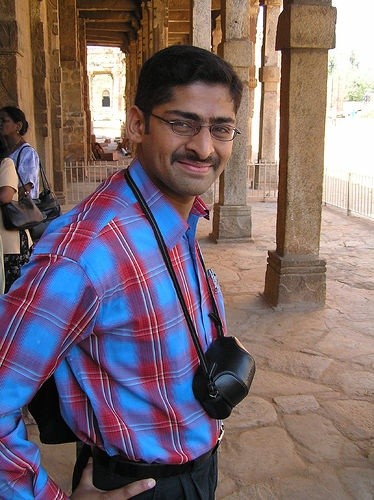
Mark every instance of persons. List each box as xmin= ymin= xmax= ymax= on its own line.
xmin=0 ymin=105 xmax=40 ymax=294
xmin=0 ymin=44 xmax=249 ymax=500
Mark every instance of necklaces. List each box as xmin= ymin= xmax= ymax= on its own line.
xmin=11 ymin=137 xmax=21 ymax=152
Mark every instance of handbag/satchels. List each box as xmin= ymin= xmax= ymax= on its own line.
xmin=2 ymin=202 xmax=43 ymax=230
xmin=31 ymin=193 xmax=62 ymax=220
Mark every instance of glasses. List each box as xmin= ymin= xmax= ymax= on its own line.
xmin=147 ymin=110 xmax=241 ymax=142
